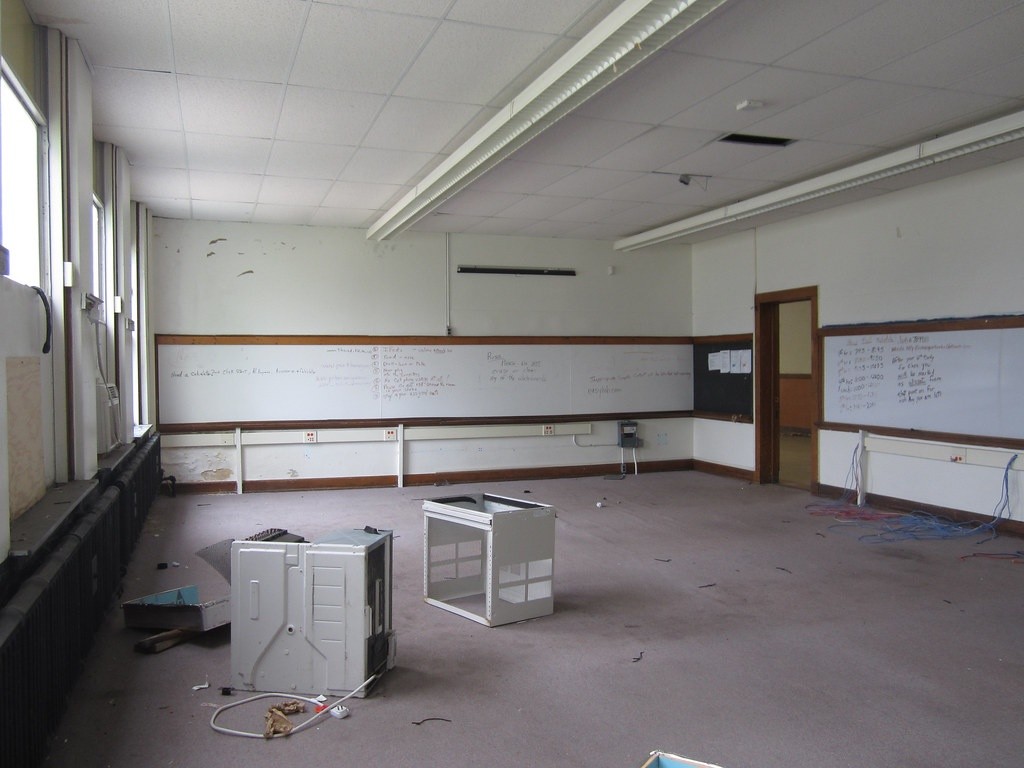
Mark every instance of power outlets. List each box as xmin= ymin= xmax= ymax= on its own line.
xmin=542 ymin=425 xmax=554 ymax=435
xmin=384 ymin=429 xmax=396 ymax=441
xmin=303 ymin=431 xmax=317 ymax=443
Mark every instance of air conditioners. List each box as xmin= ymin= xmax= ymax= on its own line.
xmin=226 ymin=529 xmax=393 ymax=700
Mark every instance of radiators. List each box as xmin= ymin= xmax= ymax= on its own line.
xmin=0 ymin=430 xmax=177 ymax=768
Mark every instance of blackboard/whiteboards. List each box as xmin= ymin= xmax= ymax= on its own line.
xmin=814 ymin=315 xmax=1024 ymax=451
xmin=154 ymin=333 xmax=755 ymax=434
